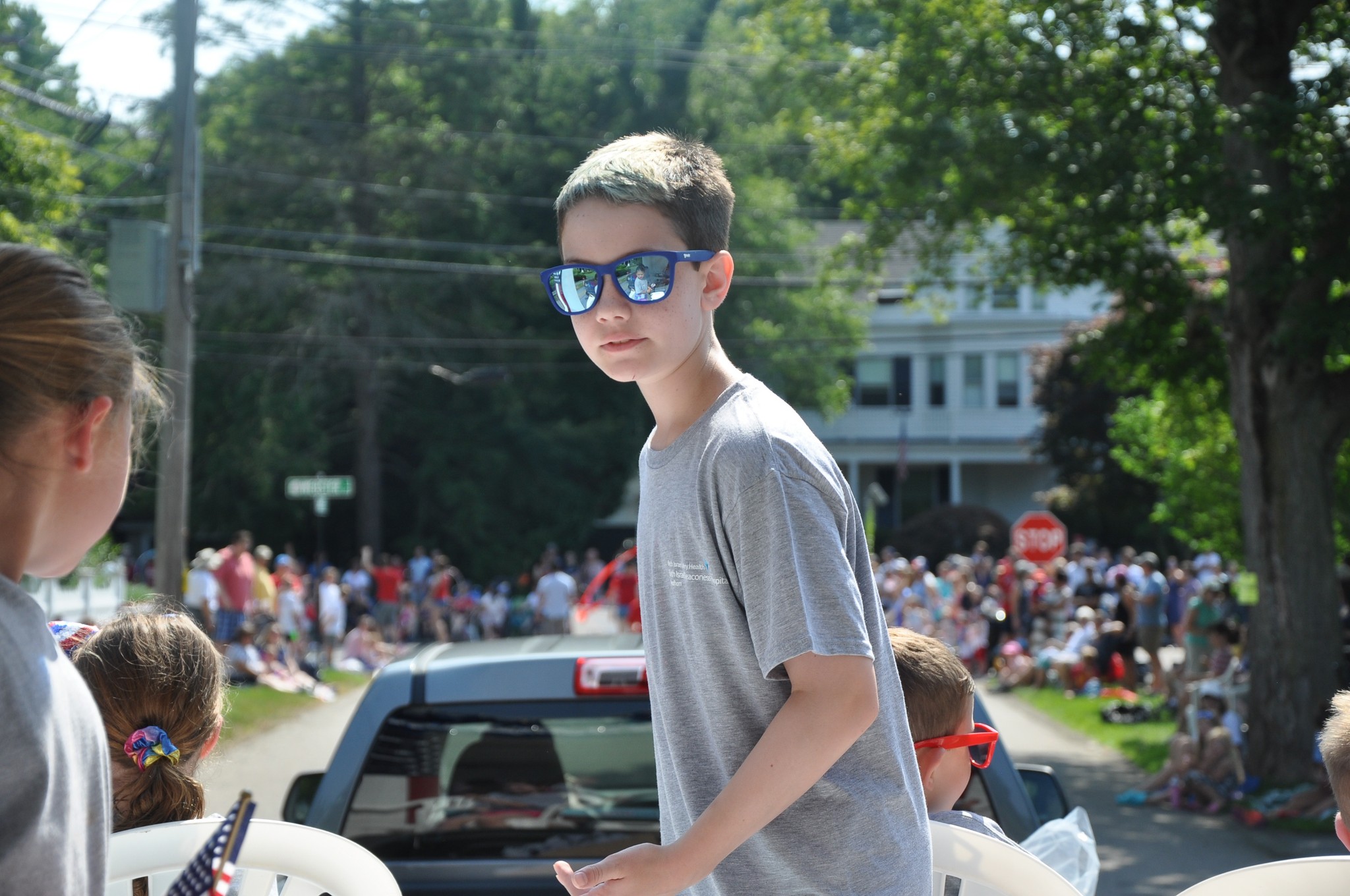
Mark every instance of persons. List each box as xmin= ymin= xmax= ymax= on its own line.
xmin=551 ymin=131 xmax=933 ymax=896
xmin=871 ymin=544 xmax=1251 ymax=815
xmin=1317 ymin=689 xmax=1349 ymax=852
xmin=186 ymin=528 xmax=607 ymax=700
xmin=72 ymin=613 xmax=224 ymax=834
xmin=0 ymin=245 xmax=139 ymax=896
xmin=878 ymin=627 xmax=1037 ymax=861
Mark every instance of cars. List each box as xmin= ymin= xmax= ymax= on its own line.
xmin=274 ymin=621 xmax=1076 ymax=896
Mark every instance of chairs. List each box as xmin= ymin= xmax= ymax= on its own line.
xmin=446 ymin=726 xmax=567 ymax=845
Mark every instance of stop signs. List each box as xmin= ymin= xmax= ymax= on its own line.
xmin=1010 ymin=509 xmax=1067 ymax=566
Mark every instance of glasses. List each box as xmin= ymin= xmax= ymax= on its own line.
xmin=540 ymin=250 xmax=713 ymax=306
xmin=914 ymin=721 xmax=1000 ymax=771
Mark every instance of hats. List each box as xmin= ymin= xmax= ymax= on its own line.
xmin=1134 ymin=551 xmax=1159 ymax=566
xmin=192 ymin=547 xmax=223 ymax=571
